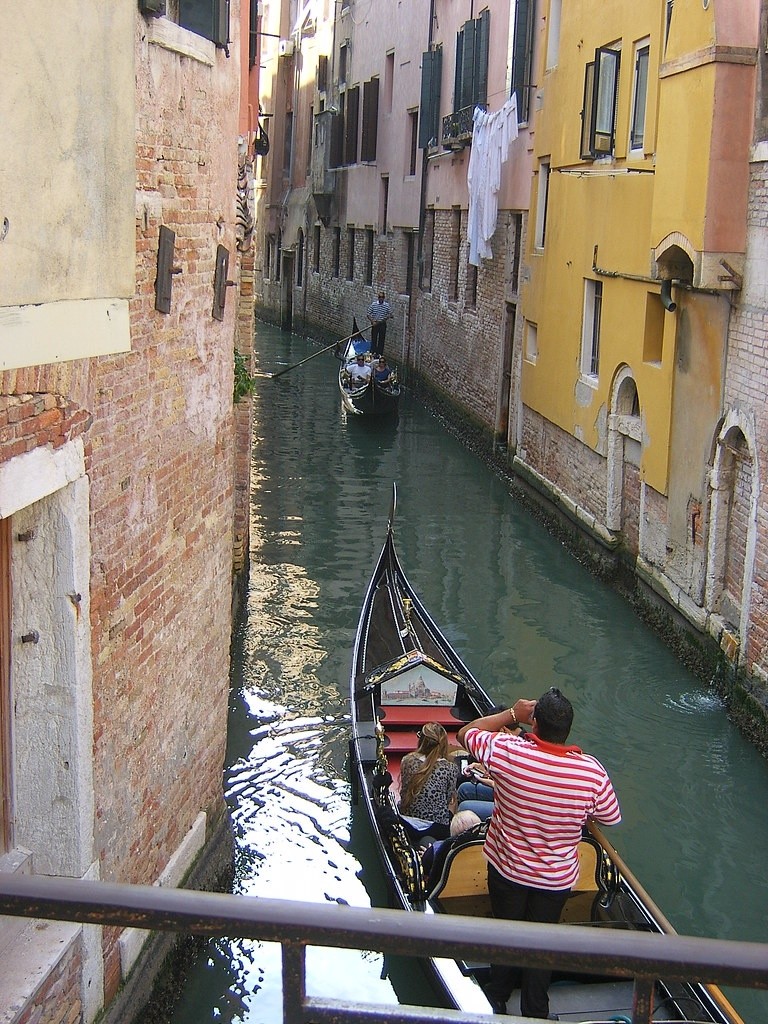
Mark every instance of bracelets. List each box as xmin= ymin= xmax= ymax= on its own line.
xmin=510 ymin=707 xmax=517 ymax=723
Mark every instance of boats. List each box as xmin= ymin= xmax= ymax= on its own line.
xmin=338 ymin=338 xmax=404 ymax=416
xmin=349 ymin=480 xmax=746 ymax=1024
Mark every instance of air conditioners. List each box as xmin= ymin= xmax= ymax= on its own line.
xmin=278 ymin=40 xmax=294 ymax=58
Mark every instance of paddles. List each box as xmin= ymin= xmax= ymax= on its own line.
xmin=273 ymin=314 xmax=391 ymax=378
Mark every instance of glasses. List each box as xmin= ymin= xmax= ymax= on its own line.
xmin=378 ymin=296 xmax=385 ymax=298
xmin=379 ymin=361 xmax=385 ymax=364
xmin=416 ymin=731 xmax=421 ymax=739
xmin=357 ymin=359 xmax=364 ymax=362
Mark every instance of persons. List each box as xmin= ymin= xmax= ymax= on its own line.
xmin=367 ymin=291 xmax=393 ymax=356
xmin=399 ymin=722 xmax=456 ymax=840
xmin=456 ymin=686 xmax=621 ymax=1020
xmin=345 ymin=355 xmax=371 ymax=392
xmin=373 ymin=357 xmax=392 ymax=393
xmin=416 ymin=704 xmax=530 ymax=885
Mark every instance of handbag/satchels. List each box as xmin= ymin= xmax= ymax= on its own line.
xmin=254 ymin=121 xmax=269 ymax=157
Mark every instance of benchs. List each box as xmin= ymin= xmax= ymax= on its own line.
xmin=433 ymin=835 xmax=652 ymax=975
xmin=376 ymin=704 xmax=466 ymax=754
